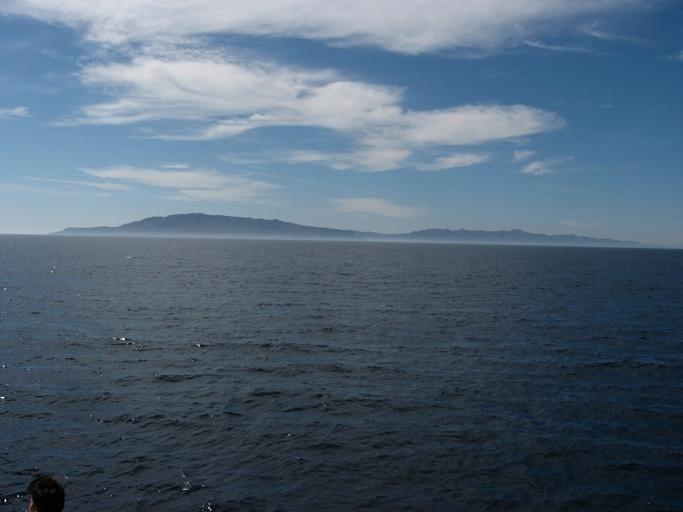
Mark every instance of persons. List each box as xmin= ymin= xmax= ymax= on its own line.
xmin=25 ymin=475 xmax=66 ymax=512
